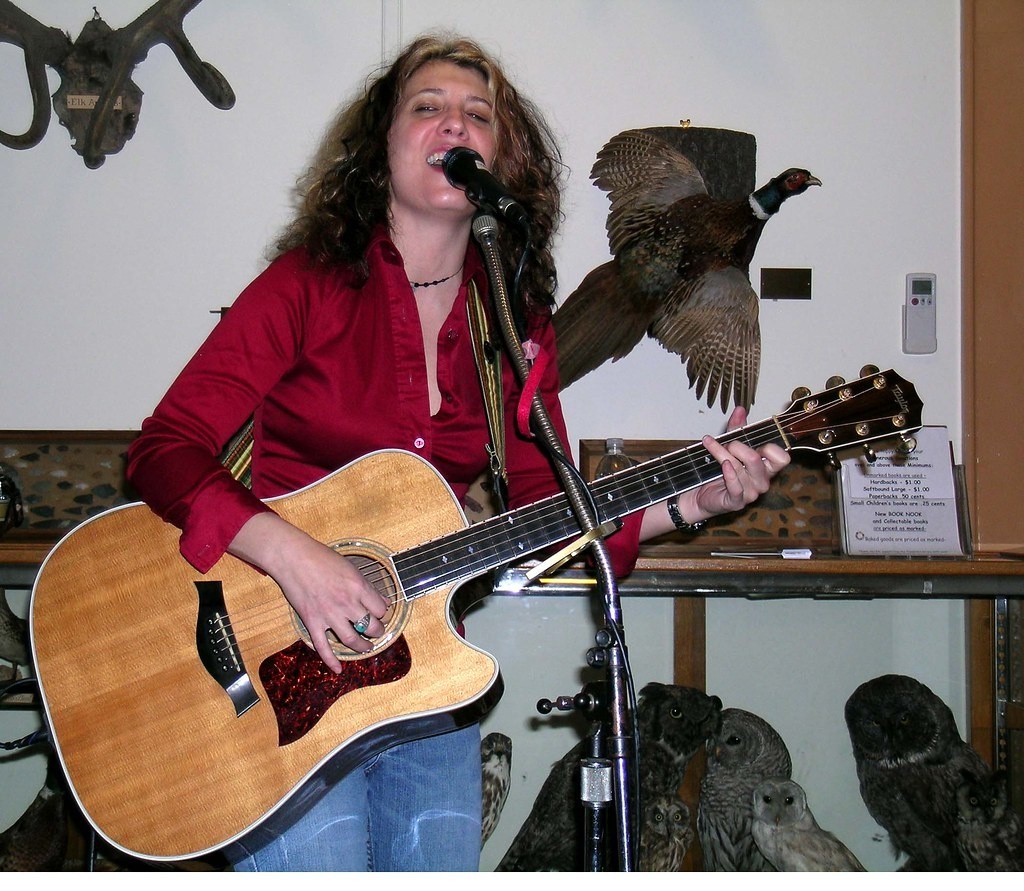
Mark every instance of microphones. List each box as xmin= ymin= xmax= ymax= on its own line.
xmin=440 ymin=146 xmax=529 ymax=224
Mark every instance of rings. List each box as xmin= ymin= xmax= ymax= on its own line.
xmin=354 ymin=613 xmax=370 ymax=633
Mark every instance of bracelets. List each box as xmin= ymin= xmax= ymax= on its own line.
xmin=668 ymin=496 xmax=707 ymax=532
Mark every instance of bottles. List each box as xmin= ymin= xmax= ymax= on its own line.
xmin=593 ymin=438 xmax=633 ymax=482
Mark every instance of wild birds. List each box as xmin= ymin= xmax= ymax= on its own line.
xmin=550 ymin=126 xmax=826 ymax=417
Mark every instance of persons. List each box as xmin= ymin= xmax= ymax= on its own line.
xmin=124 ymin=39 xmax=791 ymax=872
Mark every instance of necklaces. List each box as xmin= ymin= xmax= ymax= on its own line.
xmin=409 ymin=264 xmax=464 ymax=288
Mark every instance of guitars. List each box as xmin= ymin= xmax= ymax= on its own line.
xmin=27 ymin=362 xmax=926 ymax=872
